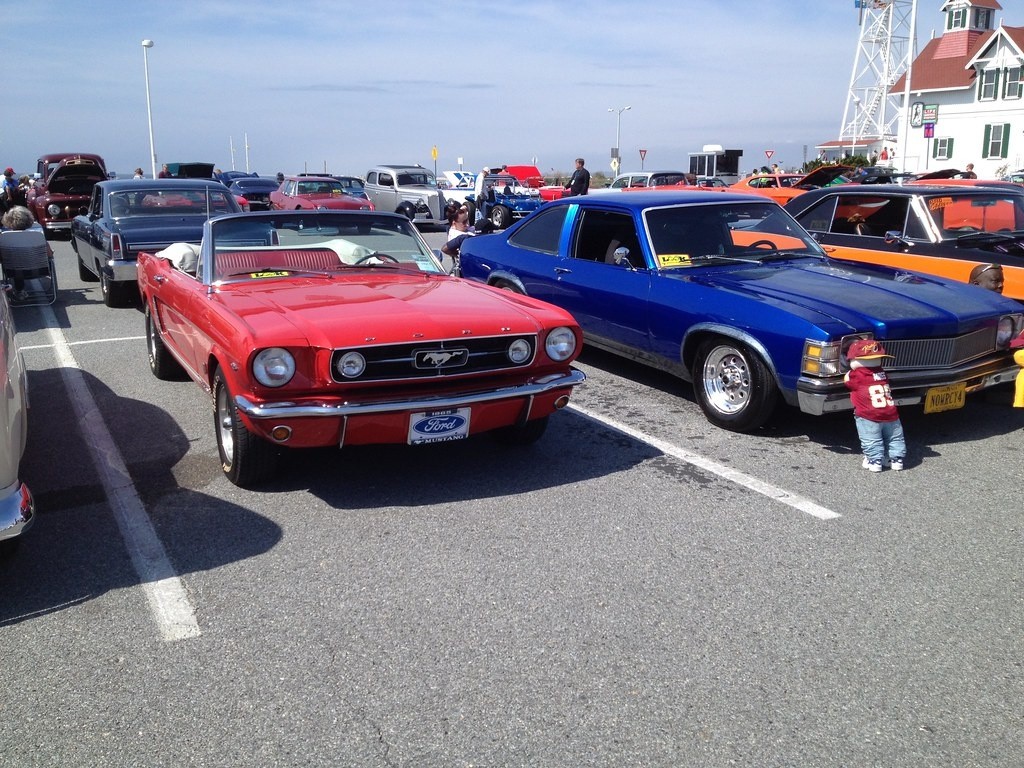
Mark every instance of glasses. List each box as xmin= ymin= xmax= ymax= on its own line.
xmin=456 ymin=217 xmax=468 ymax=225
xmin=974 ymin=263 xmax=1003 ymax=279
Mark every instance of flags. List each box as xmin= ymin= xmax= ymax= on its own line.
xmin=432 ymin=147 xmax=438 ymax=161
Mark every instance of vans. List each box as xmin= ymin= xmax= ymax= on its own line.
xmin=361 ymin=164 xmax=444 ymax=223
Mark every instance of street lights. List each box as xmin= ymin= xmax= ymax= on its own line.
xmin=608 ymin=106 xmax=631 ymax=177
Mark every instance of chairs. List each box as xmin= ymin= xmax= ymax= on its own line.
xmin=605 ymin=223 xmax=638 ymax=264
xmin=0 ymin=231 xmax=58 ymax=308
xmin=855 ymin=223 xmax=871 ymax=235
xmin=298 ymin=185 xmax=307 ymax=194
xmin=318 ymin=186 xmax=329 ymax=192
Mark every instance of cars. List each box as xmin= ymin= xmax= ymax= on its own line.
xmin=606 ymin=167 xmax=859 ymax=206
xmin=834 ymin=180 xmax=1024 ymax=233
xmin=0 ymin=263 xmax=35 ymax=540
xmin=226 ymin=178 xmax=280 ymax=213
xmin=457 ymin=191 xmax=1024 ymax=431
xmin=25 ymin=153 xmax=113 ymax=232
xmin=70 ymin=178 xmax=279 ymax=307
xmin=141 ymin=193 xmax=250 ymax=213
xmin=269 ymin=176 xmax=375 ymax=210
xmin=728 ymin=184 xmax=1024 ymax=310
xmin=440 ymin=175 xmax=571 ymax=229
xmin=135 ymin=211 xmax=587 ymax=485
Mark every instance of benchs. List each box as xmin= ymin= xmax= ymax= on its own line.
xmin=210 ymin=248 xmax=339 ymax=278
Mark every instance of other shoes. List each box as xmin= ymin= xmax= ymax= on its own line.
xmin=862 ymin=457 xmax=881 ymax=471
xmin=882 ymin=454 xmax=903 ymax=470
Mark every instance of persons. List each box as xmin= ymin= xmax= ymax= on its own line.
xmin=215 ymin=169 xmax=232 ymax=188
xmin=749 ymin=164 xmax=784 ymax=188
xmin=563 ymin=158 xmax=590 ymax=196
xmin=0 ymin=206 xmax=55 ymax=301
xmin=132 ymin=168 xmax=145 ymax=180
xmin=968 ymin=263 xmax=1004 ymax=295
xmin=962 ymin=163 xmax=977 ymax=179
xmin=817 ymin=148 xmax=837 ymax=166
xmin=843 ymin=339 xmax=907 ymax=472
xmin=159 ymin=164 xmax=172 ymax=178
xmin=858 ymin=165 xmax=869 ymax=176
xmin=474 ymin=166 xmax=491 ymax=201
xmin=276 ymin=172 xmax=285 ymax=185
xmin=0 ymin=166 xmax=36 ymax=214
xmin=498 ymin=164 xmax=509 ymax=187
xmin=441 ymin=197 xmax=499 ymax=278
xmin=872 ymin=147 xmax=895 ymax=160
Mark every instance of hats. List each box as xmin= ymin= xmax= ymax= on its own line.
xmin=483 ymin=167 xmax=490 ymax=175
xmin=847 ymin=340 xmax=895 ymax=359
xmin=4 ymin=168 xmax=16 ymax=175
